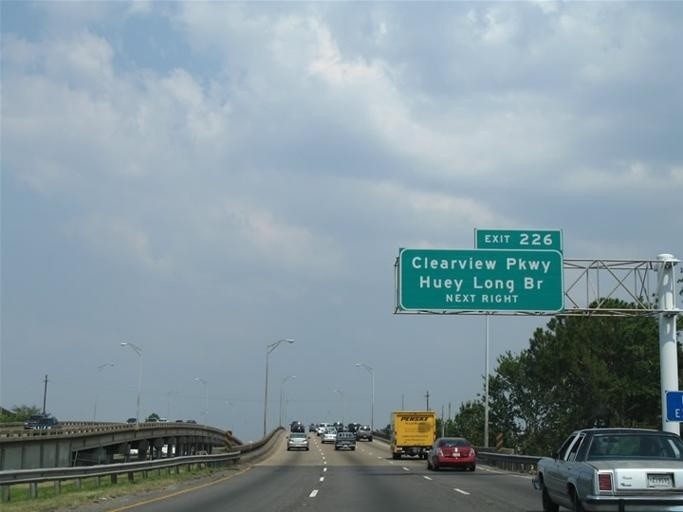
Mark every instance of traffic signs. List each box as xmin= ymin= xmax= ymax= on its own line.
xmin=475 ymin=229 xmax=563 ymax=250
xmin=396 ymin=249 xmax=564 ymax=313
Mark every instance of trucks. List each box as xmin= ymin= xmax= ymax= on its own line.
xmin=388 ymin=407 xmax=440 ymax=459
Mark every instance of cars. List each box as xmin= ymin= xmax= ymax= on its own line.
xmin=533 ymin=425 xmax=683 ymax=511
xmin=126 ymin=417 xmax=200 ymax=427
xmin=282 ymin=418 xmax=374 ymax=452
xmin=424 ymin=435 xmax=479 ymax=476
xmin=24 ymin=415 xmax=61 ymax=437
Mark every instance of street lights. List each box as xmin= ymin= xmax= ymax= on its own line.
xmin=261 ymin=337 xmax=298 ymax=436
xmin=351 ymin=359 xmax=375 ymax=435
xmin=118 ymin=339 xmax=146 ymax=430
